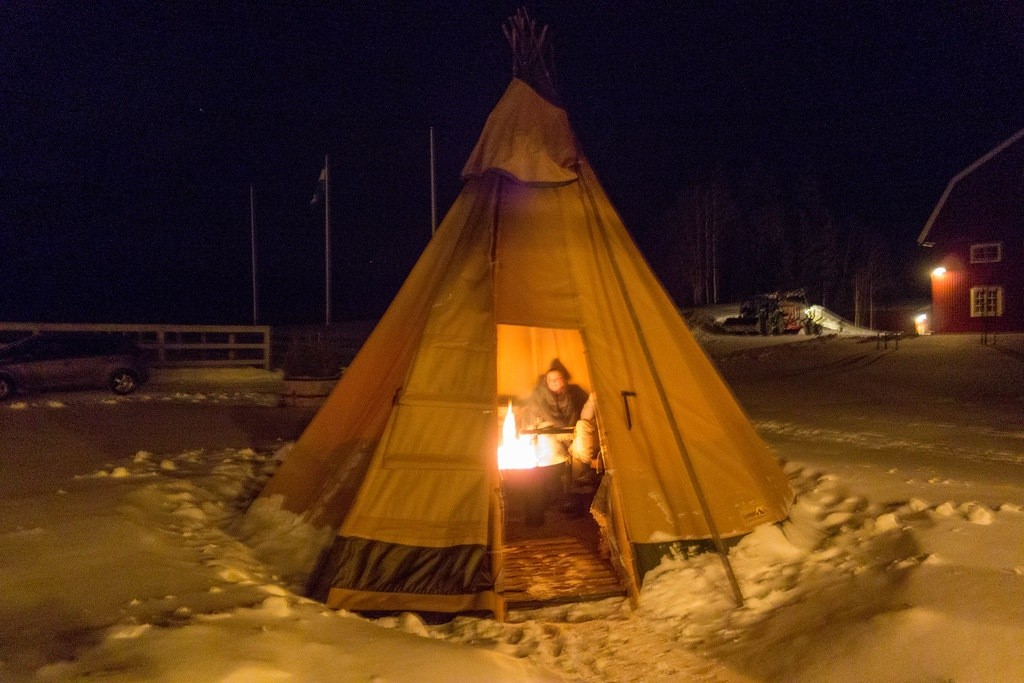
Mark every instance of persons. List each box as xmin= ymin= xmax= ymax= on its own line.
xmin=523 ymin=359 xmax=604 ymax=529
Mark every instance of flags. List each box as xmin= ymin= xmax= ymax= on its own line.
xmin=309 ymin=156 xmax=326 ymax=205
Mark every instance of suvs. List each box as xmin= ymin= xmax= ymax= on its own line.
xmin=1 ymin=330 xmax=153 ymax=401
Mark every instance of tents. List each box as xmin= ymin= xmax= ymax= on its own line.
xmin=226 ymin=75 xmax=798 ymax=628
